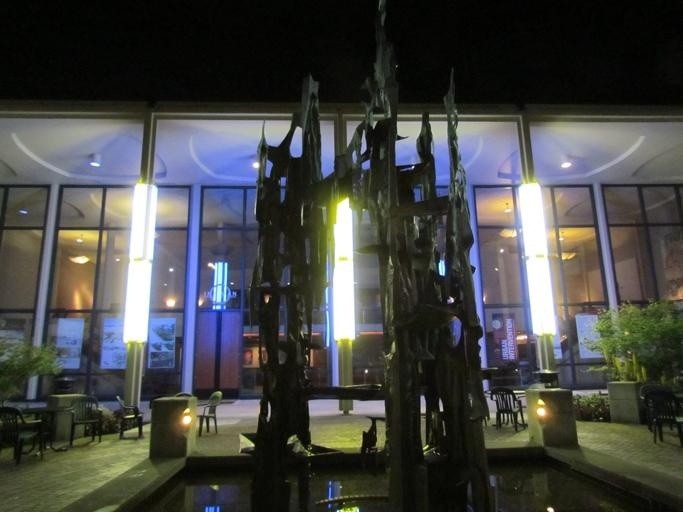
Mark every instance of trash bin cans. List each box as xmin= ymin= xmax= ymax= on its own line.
xmin=531 ymin=369 xmax=560 ymax=388
xmin=54 ymin=377 xmax=76 ymax=394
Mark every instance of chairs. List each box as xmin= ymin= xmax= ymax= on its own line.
xmin=490 ymin=386 xmax=526 ymax=431
xmin=196 ymin=389 xmax=222 ymax=436
xmin=0 ymin=393 xmax=144 ymax=464
xmin=641 ymin=382 xmax=682 ymax=449
